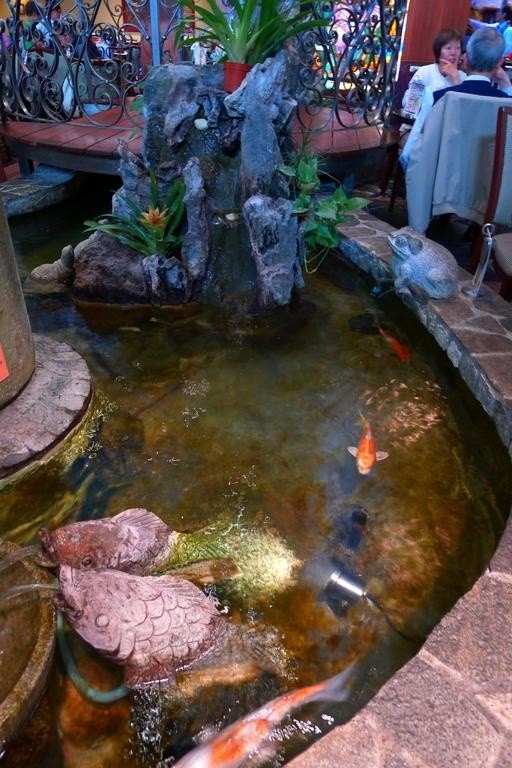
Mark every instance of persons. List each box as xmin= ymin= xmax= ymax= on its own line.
xmin=0 ymin=0 xmax=117 ymax=60
xmin=467 ymin=0 xmax=512 ymax=56
xmin=397 ymin=26 xmax=468 ymax=148
xmin=398 ymin=26 xmax=512 ymax=241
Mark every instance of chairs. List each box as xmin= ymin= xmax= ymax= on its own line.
xmin=36 ymin=42 xmax=141 ymax=115
xmin=380 ymin=60 xmax=512 ymax=302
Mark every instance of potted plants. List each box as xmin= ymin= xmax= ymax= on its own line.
xmin=173 ymin=0 xmax=332 ymax=93
